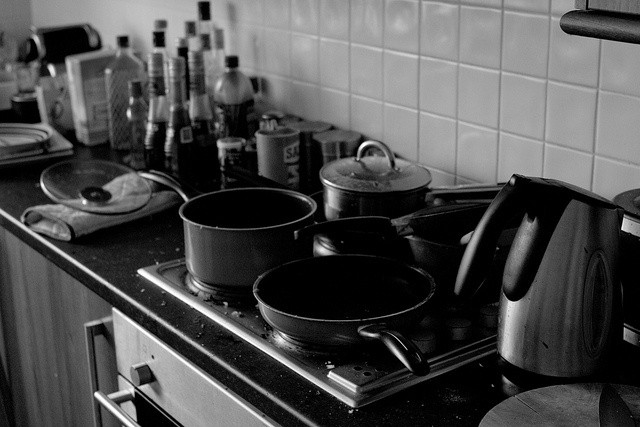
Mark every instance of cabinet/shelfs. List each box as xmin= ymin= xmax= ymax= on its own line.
xmin=1 ymin=209 xmax=118 ymax=426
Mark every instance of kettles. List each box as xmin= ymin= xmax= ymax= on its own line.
xmin=453 ymin=173 xmax=626 ymax=384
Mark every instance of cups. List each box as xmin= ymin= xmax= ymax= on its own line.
xmin=256 ymin=130 xmax=300 ymax=185
xmin=288 ymin=121 xmax=333 ymax=187
xmin=315 ymin=133 xmax=361 ymax=165
xmin=217 ymin=136 xmax=250 ymax=188
xmin=35 ymin=83 xmax=74 ymax=128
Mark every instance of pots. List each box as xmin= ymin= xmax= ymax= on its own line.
xmin=319 ymin=139 xmax=433 ymax=221
xmin=138 ymin=168 xmax=317 ymax=289
xmin=291 ymin=211 xmax=400 ymax=259
xmin=251 ymin=255 xmax=437 ymax=377
xmin=391 ymin=181 xmax=512 ymax=286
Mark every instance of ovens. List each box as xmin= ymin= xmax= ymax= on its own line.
xmin=91 ymin=305 xmax=286 ymax=427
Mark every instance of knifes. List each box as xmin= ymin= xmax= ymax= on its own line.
xmin=598 ymin=381 xmax=638 ymax=426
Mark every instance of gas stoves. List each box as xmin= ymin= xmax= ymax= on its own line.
xmin=135 ymin=257 xmax=499 ymax=410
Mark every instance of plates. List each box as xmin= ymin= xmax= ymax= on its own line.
xmin=0 ymin=148 xmax=74 ymax=165
xmin=2 ymin=123 xmax=52 ymax=155
xmin=1 ymin=120 xmax=73 ymax=164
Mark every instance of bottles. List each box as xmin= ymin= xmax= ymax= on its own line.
xmin=103 ymin=34 xmax=146 ymax=150
xmin=187 ymin=48 xmax=213 ymax=187
xmin=213 ymin=55 xmax=255 ymax=138
xmin=126 ymin=80 xmax=148 ymax=155
xmin=199 ymin=33 xmax=213 ymax=100
xmin=209 ymin=28 xmax=226 ymax=104
xmin=147 ymin=31 xmax=168 ymax=89
xmin=153 ymin=19 xmax=170 ymax=35
xmin=185 ymin=21 xmax=196 ymax=53
xmin=164 ymin=55 xmax=194 ymax=179
xmin=174 ymin=36 xmax=190 ymax=104
xmin=144 ymin=51 xmax=170 ymax=172
xmin=196 ymin=0 xmax=211 ymax=43
